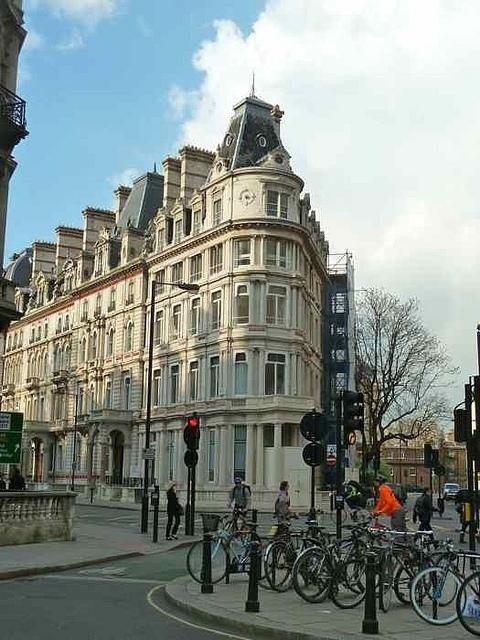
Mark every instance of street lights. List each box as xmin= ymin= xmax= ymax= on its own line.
xmin=52 ymin=390 xmax=78 ymax=491
xmin=140 ymin=281 xmax=199 ymax=534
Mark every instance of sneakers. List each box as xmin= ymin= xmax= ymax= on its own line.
xmin=166 ymin=535 xmax=177 ymax=541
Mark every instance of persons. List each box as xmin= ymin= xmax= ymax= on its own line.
xmin=412 ymin=487 xmax=442 ymax=550
xmin=225 ymin=476 xmax=252 ymax=522
xmin=272 ymin=480 xmax=290 ymax=537
xmin=9 ymin=469 xmax=26 ymax=491
xmin=369 ymin=474 xmax=406 ymax=531
xmin=453 ymin=494 xmax=480 ymax=545
xmin=165 ymin=479 xmax=180 ymax=540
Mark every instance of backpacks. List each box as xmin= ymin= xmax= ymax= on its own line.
xmin=233 ymin=485 xmax=251 ymax=499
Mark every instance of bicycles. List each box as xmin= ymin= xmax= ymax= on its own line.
xmin=186 ymin=504 xmax=480 ymax=638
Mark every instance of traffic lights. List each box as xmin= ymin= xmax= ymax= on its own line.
xmin=187 ymin=416 xmax=200 ymax=426
xmin=342 ymin=389 xmax=364 ymax=434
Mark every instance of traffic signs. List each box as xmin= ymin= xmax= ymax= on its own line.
xmin=0 ymin=411 xmax=24 ymax=466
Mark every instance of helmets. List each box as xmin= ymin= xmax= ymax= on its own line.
xmin=234 ymin=477 xmax=241 ymax=483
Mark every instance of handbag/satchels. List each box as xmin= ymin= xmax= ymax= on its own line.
xmin=176 ymin=506 xmax=184 ymax=515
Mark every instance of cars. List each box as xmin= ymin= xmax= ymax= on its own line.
xmin=442 ymin=482 xmax=461 ymax=501
